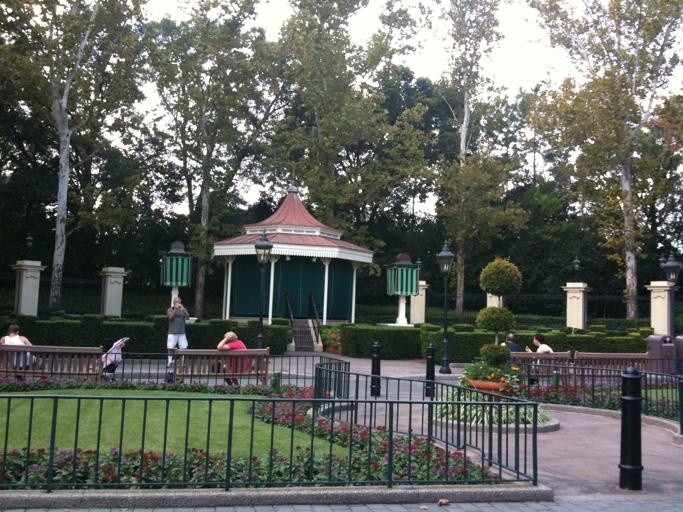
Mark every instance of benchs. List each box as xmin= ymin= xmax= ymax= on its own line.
xmin=0 ymin=328 xmax=101 ymax=393
xmin=494 ymin=344 xmax=572 ymax=385
xmin=572 ymin=352 xmax=651 ymax=386
xmin=163 ymin=345 xmax=271 ymax=394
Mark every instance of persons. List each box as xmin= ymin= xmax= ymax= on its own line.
xmin=166 ymin=297 xmax=189 ymax=367
xmin=500 ymin=333 xmax=554 ymax=385
xmin=0 ymin=324 xmax=43 ymax=370
xmin=217 ymin=331 xmax=250 ymax=386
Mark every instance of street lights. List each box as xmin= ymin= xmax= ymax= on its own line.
xmin=435 ymin=238 xmax=456 ymax=374
xmin=255 ymin=226 xmax=272 ymax=369
xmin=660 ymin=252 xmax=681 ymax=337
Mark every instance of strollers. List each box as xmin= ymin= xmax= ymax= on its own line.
xmin=92 ymin=337 xmax=130 ymax=380
